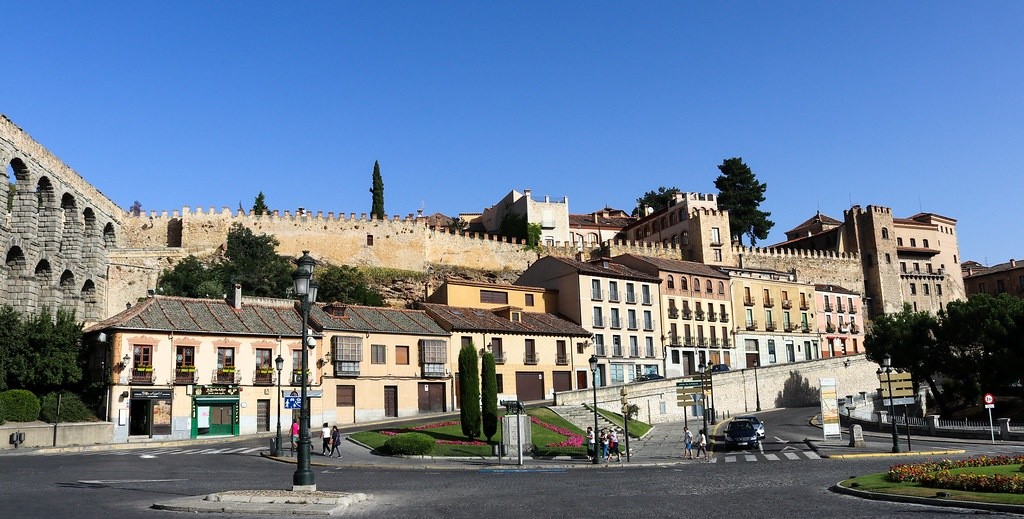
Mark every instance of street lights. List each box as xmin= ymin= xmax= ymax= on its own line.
xmin=275 ymin=355 xmax=285 ymax=457
xmin=588 ymin=354 xmax=603 ymax=464
xmin=698 ymin=362 xmax=711 ymax=448
xmin=291 ymin=249 xmax=318 ymax=492
xmin=707 ymin=360 xmax=717 ymax=424
xmin=883 ymin=352 xmax=900 ymax=453
xmin=752 ymin=358 xmax=762 ymax=412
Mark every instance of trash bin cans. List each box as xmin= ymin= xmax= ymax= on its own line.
xmin=705 ymin=409 xmax=712 ymax=420
xmin=12 ymin=433 xmax=18 ymax=442
xmin=501 ymin=444 xmax=509 ymax=455
xmin=270 ymin=438 xmax=277 ymax=456
xmin=19 ymin=433 xmax=25 ymax=441
xmin=492 ymin=444 xmax=499 ymax=456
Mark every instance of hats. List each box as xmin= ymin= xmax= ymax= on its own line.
xmin=610 ymin=427 xmax=615 ymax=432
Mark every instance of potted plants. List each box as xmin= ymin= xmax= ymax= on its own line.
xmin=260 ymin=368 xmax=274 ymax=371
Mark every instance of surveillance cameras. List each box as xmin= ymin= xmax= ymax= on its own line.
xmin=308 ymin=338 xmax=316 ymax=349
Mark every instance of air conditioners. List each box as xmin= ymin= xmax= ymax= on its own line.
xmin=294 ymin=374 xmax=302 ymax=383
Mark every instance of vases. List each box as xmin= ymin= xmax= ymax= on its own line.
xmin=297 ymin=371 xmax=309 ymax=374
xmin=223 ymin=369 xmax=235 ymax=372
xmin=261 ymin=370 xmax=273 ymax=373
xmin=137 ymin=368 xmax=152 ymax=371
xmin=181 ymin=369 xmax=196 ymax=372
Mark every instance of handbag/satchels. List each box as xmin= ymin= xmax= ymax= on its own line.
xmin=609 ymin=441 xmax=614 ymax=448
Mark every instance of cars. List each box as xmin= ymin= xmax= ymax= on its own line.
xmin=724 ymin=419 xmax=760 ymax=451
xmin=713 ymin=363 xmax=729 ymax=372
xmin=729 ymin=416 xmax=765 ymax=439
xmin=631 ymin=374 xmax=664 ymax=383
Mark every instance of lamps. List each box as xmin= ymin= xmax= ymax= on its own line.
xmin=730 ymin=325 xmax=741 ymax=335
xmin=588 ymin=335 xmax=595 ymax=348
xmin=323 ymin=352 xmax=333 ymax=365
xmin=485 ymin=342 xmax=493 ymax=354
xmin=121 ymin=354 xmax=131 ymax=370
xmin=661 ymin=330 xmax=672 ymax=340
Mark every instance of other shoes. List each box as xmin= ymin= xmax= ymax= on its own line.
xmin=688 ymin=457 xmax=693 ymax=459
xmin=615 ymin=460 xmax=622 ymax=463
xmin=703 ymin=457 xmax=708 ymax=460
xmin=329 ymin=455 xmax=332 ymax=458
xmin=585 ymin=460 xmax=591 ymax=462
xmin=605 ymin=459 xmax=610 ymax=462
xmin=695 ymin=456 xmax=699 ymax=458
xmin=320 ymin=453 xmax=326 ymax=456
xmin=337 ymin=455 xmax=342 ymax=459
xmin=329 ymin=451 xmax=332 ymax=455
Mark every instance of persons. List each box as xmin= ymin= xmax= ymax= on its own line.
xmin=603 ymin=426 xmax=622 ymax=463
xmin=599 ymin=429 xmax=612 ymax=461
xmin=320 ymin=422 xmax=331 ymax=455
xmin=307 ymin=425 xmax=314 ymax=451
xmin=329 ymin=425 xmax=343 ymax=459
xmin=694 ymin=429 xmax=709 ymax=460
xmin=682 ymin=427 xmax=694 ymax=460
xmin=288 ymin=418 xmax=300 ymax=451
xmin=585 ymin=427 xmax=595 ymax=462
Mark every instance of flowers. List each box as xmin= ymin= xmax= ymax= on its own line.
xmin=136 ymin=364 xmax=152 ymax=368
xmin=181 ymin=365 xmax=195 ymax=369
xmin=297 ymin=368 xmax=310 ymax=372
xmin=222 ymin=366 xmax=235 ymax=370
